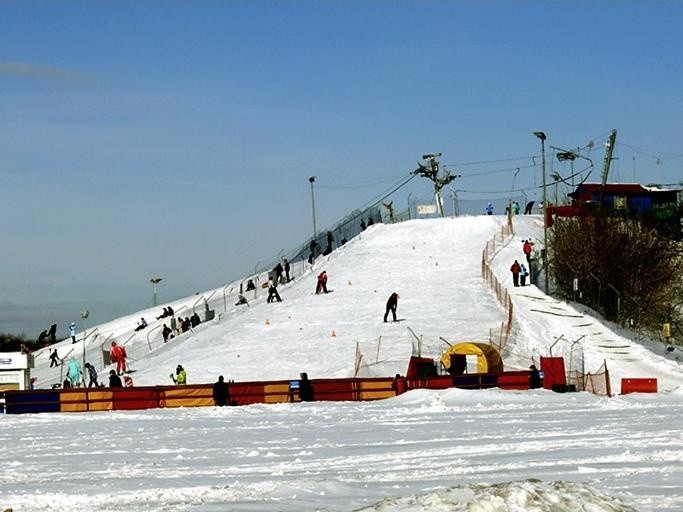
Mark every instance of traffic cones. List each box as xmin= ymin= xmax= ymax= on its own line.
xmin=331 ymin=329 xmax=337 ymax=338
xmin=265 ymin=318 xmax=271 ymax=325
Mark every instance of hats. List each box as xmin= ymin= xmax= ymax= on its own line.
xmin=529 ymin=364 xmax=536 ymax=370
xmin=123 ymin=373 xmax=129 ymax=377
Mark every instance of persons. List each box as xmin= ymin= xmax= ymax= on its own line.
xmin=307 ymin=231 xmax=334 ymax=264
xmin=235 ymin=260 xmax=290 ymax=305
xmin=485 ymin=202 xmax=493 ymax=214
xmin=510 ymin=238 xmax=535 ymax=286
xmin=315 ymin=271 xmax=328 ymax=294
xmin=212 ymin=376 xmax=230 ymax=405
xmin=298 ymin=373 xmax=313 ymax=401
xmin=359 ymin=216 xmax=374 ymax=231
xmin=528 ymin=365 xmax=541 ymax=389
xmin=36 ymin=306 xmax=201 ymax=389
xmin=391 ymin=374 xmax=408 ymax=396
xmin=384 ymin=293 xmax=398 ymax=322
xmin=504 ymin=198 xmax=535 ymax=215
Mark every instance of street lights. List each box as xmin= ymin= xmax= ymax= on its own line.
xmin=510 ymin=167 xmax=521 ymax=199
xmin=309 ymin=175 xmax=318 ymax=238
xmin=531 ymin=131 xmax=552 ymax=299
xmin=77 ymin=307 xmax=91 ymax=376
xmin=148 ymin=276 xmax=164 ymax=309
